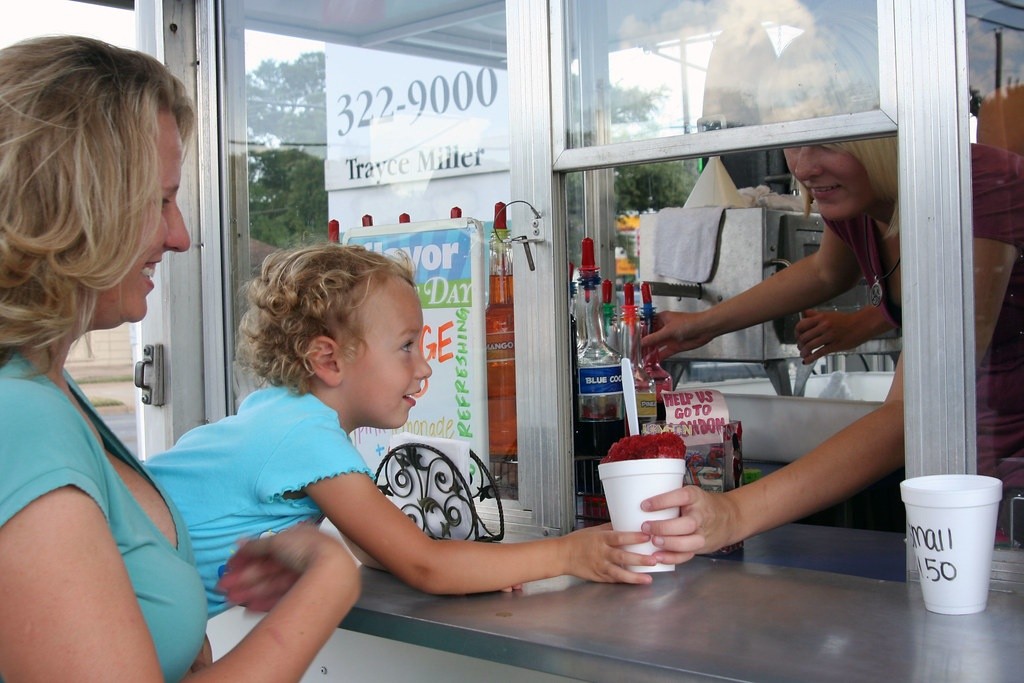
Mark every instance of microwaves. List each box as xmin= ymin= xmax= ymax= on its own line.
xmin=635 ymin=206 xmax=903 ymax=361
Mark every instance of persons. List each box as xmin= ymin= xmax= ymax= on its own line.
xmin=138 ymin=242 xmax=657 ymax=621
xmin=1 ymin=35 xmax=364 ymax=683
xmin=639 ymin=10 xmax=1024 ymax=566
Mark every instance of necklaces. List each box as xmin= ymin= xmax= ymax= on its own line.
xmin=862 ymin=214 xmax=899 ymax=308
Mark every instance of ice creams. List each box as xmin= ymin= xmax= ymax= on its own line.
xmin=599 ymin=432 xmax=687 ymax=464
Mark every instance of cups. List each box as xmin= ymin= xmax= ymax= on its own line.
xmin=597 ymin=457 xmax=686 ymax=574
xmin=899 ymin=474 xmax=1002 ymax=616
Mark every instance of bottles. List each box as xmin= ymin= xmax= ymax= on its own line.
xmin=485 ymin=229 xmax=516 ymax=462
xmin=570 ymin=268 xmax=626 ymax=497
xmin=618 ymin=305 xmax=656 ymax=436
xmin=634 ymin=307 xmax=672 ymax=426
